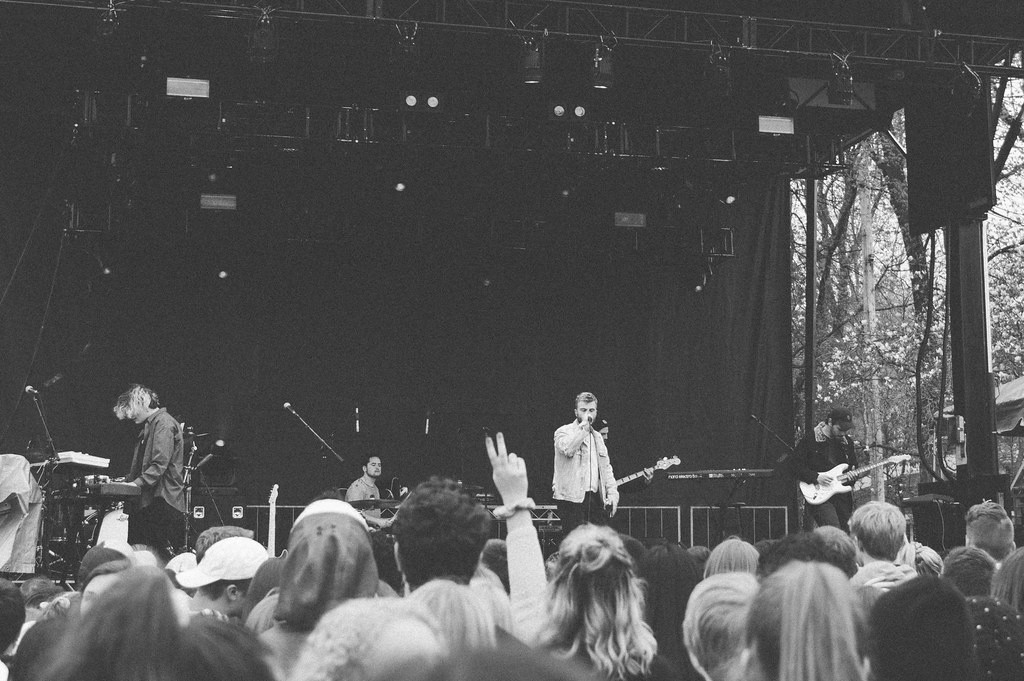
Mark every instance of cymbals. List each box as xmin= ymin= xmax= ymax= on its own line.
xmin=346 ymin=498 xmax=401 ymax=509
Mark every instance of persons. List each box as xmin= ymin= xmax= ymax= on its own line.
xmin=345 ymin=454 xmax=394 ymax=533
xmin=553 ymin=393 xmax=620 ymax=536
xmin=113 ymin=384 xmax=187 ymax=549
xmin=591 ymin=417 xmax=654 ymax=523
xmin=787 ymin=408 xmax=859 ymax=535
xmin=0 ymin=432 xmax=1024 ymax=681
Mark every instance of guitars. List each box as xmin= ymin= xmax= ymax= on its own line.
xmin=617 ymin=455 xmax=681 ymax=486
xmin=799 ymin=452 xmax=912 ymax=505
xmin=267 ymin=484 xmax=288 ymax=560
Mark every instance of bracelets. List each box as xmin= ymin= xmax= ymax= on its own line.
xmin=492 ymin=497 xmax=536 ymax=520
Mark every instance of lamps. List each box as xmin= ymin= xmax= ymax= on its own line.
xmin=94 ymin=0 xmax=983 ymax=292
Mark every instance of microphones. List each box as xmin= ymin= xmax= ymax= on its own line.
xmin=284 ymin=402 xmax=296 ymax=416
xmin=25 ymin=386 xmax=39 ymax=394
xmin=425 ymin=411 xmax=431 ymax=434
xmin=588 ymin=418 xmax=592 ymax=422
xmin=356 ymin=403 xmax=359 ymax=433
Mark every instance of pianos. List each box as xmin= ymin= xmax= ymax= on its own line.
xmin=665 ymin=468 xmax=776 ymax=549
xmin=50 ymin=451 xmax=143 ymax=594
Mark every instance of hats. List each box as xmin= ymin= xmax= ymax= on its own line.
xmin=831 ymin=407 xmax=855 ymax=430
xmin=175 ymin=537 xmax=269 ymax=589
xmin=591 ymin=416 xmax=609 ymax=432
xmin=290 ymin=499 xmax=370 ymax=534
xmin=19 ymin=577 xmax=63 ymax=605
